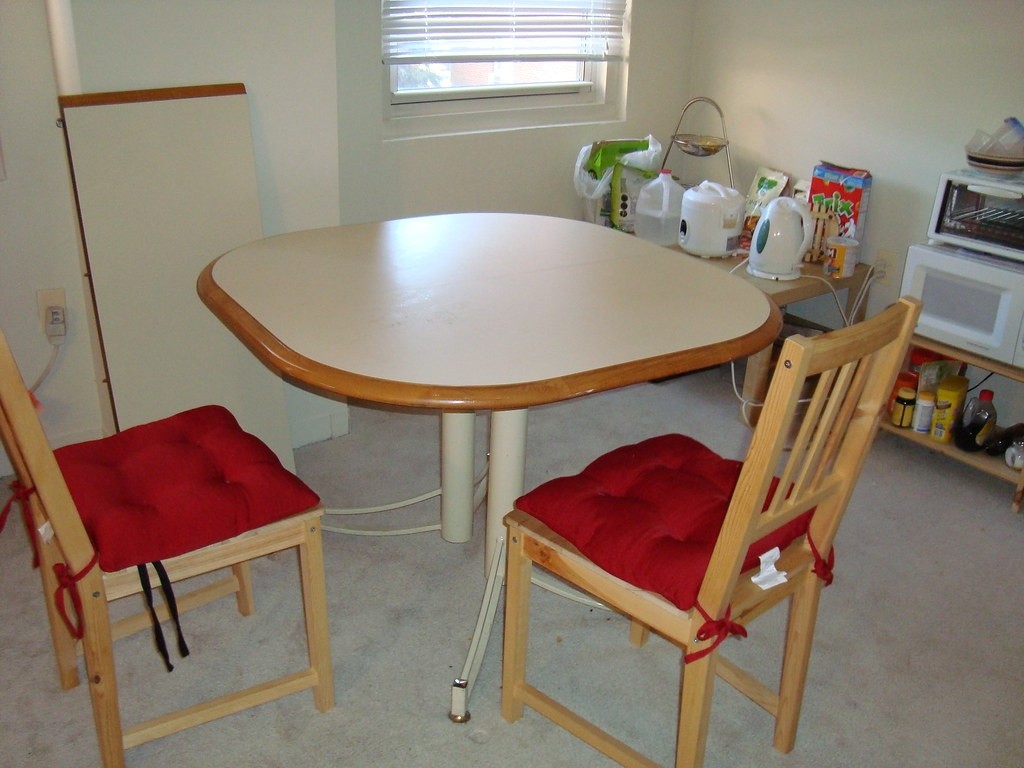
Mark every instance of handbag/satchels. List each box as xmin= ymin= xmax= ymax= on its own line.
xmin=573 ymin=134 xmax=663 ymax=230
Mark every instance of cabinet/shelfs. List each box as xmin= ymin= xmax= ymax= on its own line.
xmin=893 ymin=336 xmax=1024 ymax=512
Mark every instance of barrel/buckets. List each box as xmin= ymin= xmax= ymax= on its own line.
xmin=823 ymin=237 xmax=860 ymax=279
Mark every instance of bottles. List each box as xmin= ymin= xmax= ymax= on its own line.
xmin=892 ymin=386 xmax=916 ymax=428
xmin=912 ymin=391 xmax=936 ymax=434
xmin=633 ymin=168 xmax=685 ymax=250
xmin=955 ymin=388 xmax=997 ymax=452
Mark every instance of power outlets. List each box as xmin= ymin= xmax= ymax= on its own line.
xmin=873 ymin=249 xmax=896 ymax=286
xmin=36 ymin=290 xmax=65 ymax=332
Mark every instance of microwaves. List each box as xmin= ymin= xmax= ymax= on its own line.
xmin=897 ymin=239 xmax=1024 ymax=370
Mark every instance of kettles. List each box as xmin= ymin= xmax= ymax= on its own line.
xmin=745 ymin=196 xmax=814 ymax=282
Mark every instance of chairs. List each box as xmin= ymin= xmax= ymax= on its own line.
xmin=501 ymin=298 xmax=924 ymax=768
xmin=0 ymin=338 xmax=335 ymax=768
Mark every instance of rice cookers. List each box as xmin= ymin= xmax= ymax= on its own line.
xmin=678 ymin=180 xmax=746 ymax=260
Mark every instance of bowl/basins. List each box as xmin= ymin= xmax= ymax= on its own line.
xmin=964 ymin=117 xmax=1024 ymax=179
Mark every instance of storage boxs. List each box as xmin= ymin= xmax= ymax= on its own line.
xmin=811 ymin=160 xmax=873 ymax=265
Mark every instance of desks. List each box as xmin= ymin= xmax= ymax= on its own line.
xmin=611 ymin=225 xmax=875 ymax=426
xmin=192 ymin=214 xmax=783 ymax=719
